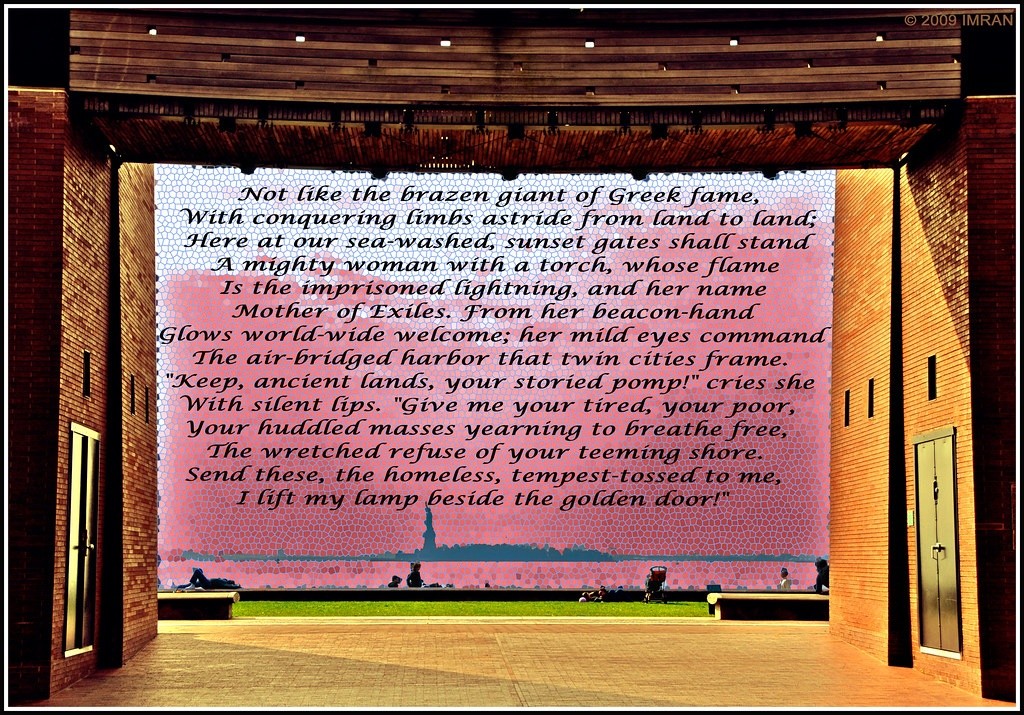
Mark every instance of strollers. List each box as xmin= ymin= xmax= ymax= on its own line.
xmin=643 ymin=565 xmax=668 ymax=605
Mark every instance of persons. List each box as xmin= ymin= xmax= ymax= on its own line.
xmin=407 ymin=563 xmax=422 ymax=587
xmin=177 ymin=569 xmax=240 ymax=589
xmin=579 ymin=586 xmax=624 ymax=602
xmin=158 ymin=555 xmax=161 ymax=587
xmin=389 ymin=576 xmax=398 ymax=588
xmin=814 ymin=557 xmax=829 ymax=595
xmin=780 ymin=568 xmax=790 ymax=591
xmin=644 ymin=575 xmax=649 ymax=592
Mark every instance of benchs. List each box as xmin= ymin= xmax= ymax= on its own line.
xmin=158 ymin=592 xmax=240 ymax=620
xmin=707 ymin=593 xmax=829 ymax=619
